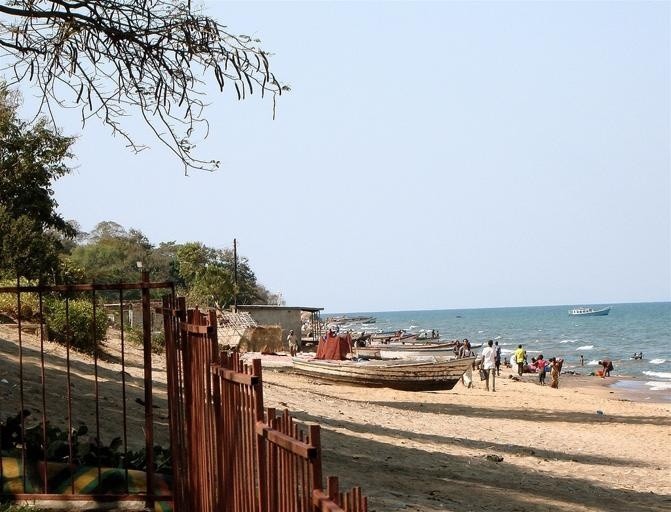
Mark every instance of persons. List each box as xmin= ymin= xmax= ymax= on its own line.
xmin=287 ymin=330 xmax=299 ymax=357
xmin=437 ymin=331 xmax=439 ymax=337
xmin=631 ymin=353 xmax=638 ymax=360
xmin=454 ymin=338 xmax=559 ymax=392
xmin=432 ymin=330 xmax=436 ymax=338
xmin=380 ymin=329 xmax=384 ymax=333
xmin=580 ymin=355 xmax=584 ymax=366
xmin=417 ymin=332 xmax=428 ymax=339
xmin=599 ymin=360 xmax=614 ymax=378
xmin=302 ymin=323 xmax=306 ymax=331
xmin=638 ymin=352 xmax=642 ymax=359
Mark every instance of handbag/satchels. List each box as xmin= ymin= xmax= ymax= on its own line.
xmin=479 ymin=369 xmax=488 ymax=381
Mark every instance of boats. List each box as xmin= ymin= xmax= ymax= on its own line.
xmin=567 ymin=300 xmax=611 ymax=316
xmin=240 ymin=311 xmax=485 ymax=395
